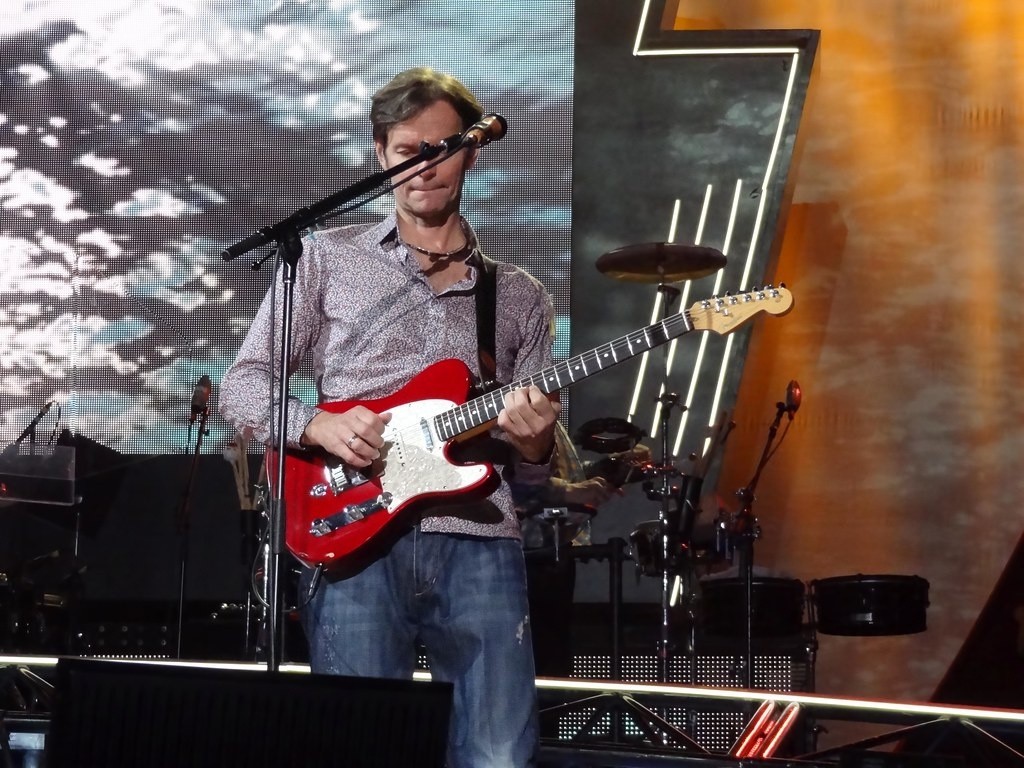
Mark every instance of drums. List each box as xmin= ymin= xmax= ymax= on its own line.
xmin=630 ymin=520 xmax=935 ymax=635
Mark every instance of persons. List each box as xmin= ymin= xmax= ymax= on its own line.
xmin=216 ymin=66 xmax=565 ymax=768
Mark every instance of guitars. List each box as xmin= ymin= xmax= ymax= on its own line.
xmin=267 ymin=280 xmax=796 ymax=573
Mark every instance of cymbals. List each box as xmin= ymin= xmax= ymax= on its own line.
xmin=594 ymin=242 xmax=727 ymax=283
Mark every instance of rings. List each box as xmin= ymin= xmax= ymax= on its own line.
xmin=347 ymin=434 xmax=359 ymax=449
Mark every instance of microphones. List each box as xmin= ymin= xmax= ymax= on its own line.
xmin=786 ymin=380 xmax=802 ymax=420
xmin=191 ymin=375 xmax=210 ymax=419
xmin=4 ymin=403 xmax=52 ymax=454
xmin=464 ymin=112 xmax=508 ymax=148
